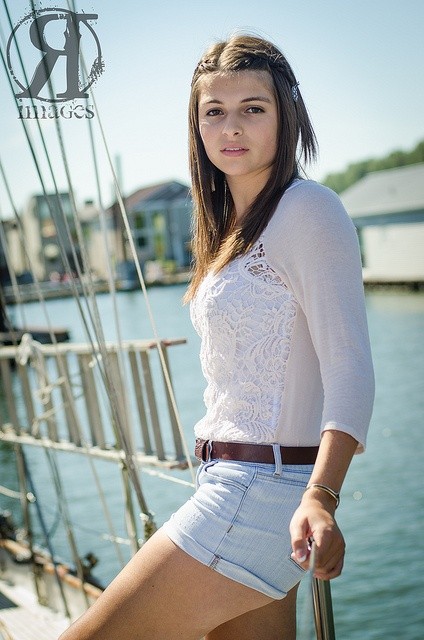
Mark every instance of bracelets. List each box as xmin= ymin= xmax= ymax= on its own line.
xmin=305 ymin=483 xmax=340 ymax=508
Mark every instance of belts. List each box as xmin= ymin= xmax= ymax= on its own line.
xmin=194 ymin=437 xmax=320 ymax=465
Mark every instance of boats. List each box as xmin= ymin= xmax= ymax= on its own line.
xmin=0 ymin=0 xmax=337 ymax=640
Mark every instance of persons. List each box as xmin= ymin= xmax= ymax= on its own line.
xmin=57 ymin=34 xmax=376 ymax=640
